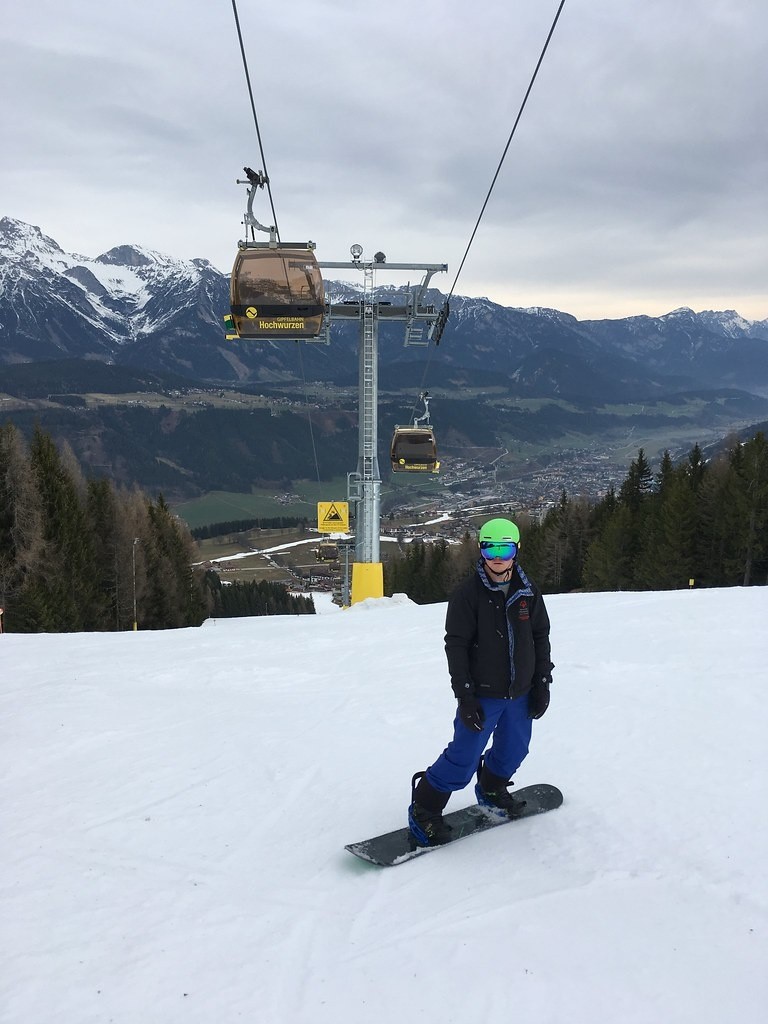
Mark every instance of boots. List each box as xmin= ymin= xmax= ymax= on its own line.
xmin=478 ymin=764 xmax=527 ymax=819
xmin=408 ymin=773 xmax=453 ymax=846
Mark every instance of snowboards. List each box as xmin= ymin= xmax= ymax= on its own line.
xmin=345 ymin=756 xmax=563 ymax=867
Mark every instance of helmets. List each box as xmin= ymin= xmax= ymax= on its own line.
xmin=479 ymin=518 xmax=521 ymax=545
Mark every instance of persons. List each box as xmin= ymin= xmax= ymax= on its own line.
xmin=405 ymin=516 xmax=556 ymax=849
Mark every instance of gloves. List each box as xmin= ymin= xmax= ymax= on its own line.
xmin=458 ymin=694 xmax=485 ymax=733
xmin=525 ymin=682 xmax=550 ymax=720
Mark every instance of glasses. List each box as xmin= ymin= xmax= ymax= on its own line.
xmin=479 ymin=541 xmax=517 ymax=561
